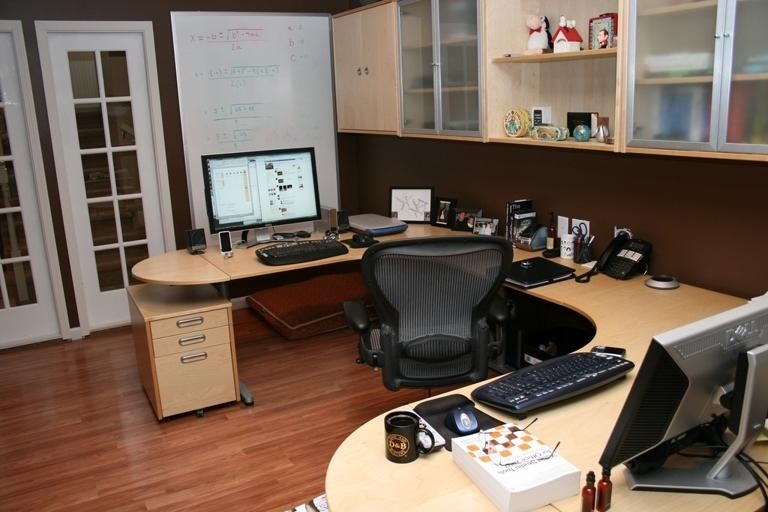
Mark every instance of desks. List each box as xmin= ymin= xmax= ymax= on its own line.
xmin=130 ymin=218 xmax=767 ymax=512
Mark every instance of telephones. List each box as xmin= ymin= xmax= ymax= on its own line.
xmin=571 ymin=223 xmax=587 ymax=241
xmin=596 ymin=237 xmax=652 ymax=279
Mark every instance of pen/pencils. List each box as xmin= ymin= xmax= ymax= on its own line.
xmin=577 ymin=232 xmax=595 ymax=262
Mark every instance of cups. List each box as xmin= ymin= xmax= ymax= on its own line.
xmin=385 ymin=410 xmax=435 ymax=464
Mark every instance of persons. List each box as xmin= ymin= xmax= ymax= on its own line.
xmin=439 ymin=203 xmax=496 ymax=236
xmin=424 ymin=213 xmax=430 ymax=221
xmin=392 ymin=213 xmax=397 ymax=219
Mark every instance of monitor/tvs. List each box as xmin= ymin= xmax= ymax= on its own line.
xmin=201 ymin=147 xmax=322 ymax=248
xmin=598 ymin=294 xmax=768 ymax=500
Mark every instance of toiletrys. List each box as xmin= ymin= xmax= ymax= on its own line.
xmin=597 ymin=466 xmax=612 ymax=512
xmin=581 ymin=470 xmax=596 ymax=512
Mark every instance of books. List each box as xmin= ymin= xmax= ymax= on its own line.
xmin=448 ymin=420 xmax=581 ymax=512
xmin=504 ymin=197 xmax=538 ymax=247
xmin=641 ymin=50 xmax=768 ymax=143
xmin=487 ymin=256 xmax=577 ymax=290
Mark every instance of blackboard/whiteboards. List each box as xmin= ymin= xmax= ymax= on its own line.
xmin=171 ymin=11 xmax=342 ymax=247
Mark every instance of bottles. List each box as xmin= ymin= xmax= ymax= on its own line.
xmin=545 ymin=212 xmax=557 ymax=251
xmin=560 ymin=233 xmax=576 ymax=259
xmin=533 ymin=109 xmax=542 ymax=125
xmin=482 ymin=223 xmax=494 ymax=235
xmin=596 ymin=124 xmax=609 ymax=142
xmin=440 ymin=203 xmax=447 ymax=222
xmin=580 ymin=466 xmax=614 ymax=512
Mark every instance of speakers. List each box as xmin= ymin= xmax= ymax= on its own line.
xmin=184 ymin=228 xmax=206 ymax=255
xmin=337 ymin=210 xmax=350 ymax=234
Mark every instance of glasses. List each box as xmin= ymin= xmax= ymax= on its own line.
xmin=478 ymin=419 xmax=560 ymax=464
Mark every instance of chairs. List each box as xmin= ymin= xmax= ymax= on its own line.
xmin=342 ymin=235 xmax=514 ymax=397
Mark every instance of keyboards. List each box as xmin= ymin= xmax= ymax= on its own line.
xmin=471 ymin=352 xmax=635 ymax=415
xmin=255 ymin=239 xmax=348 ymax=265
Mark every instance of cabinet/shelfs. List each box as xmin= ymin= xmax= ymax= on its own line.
xmin=332 ymin=0 xmax=400 ymax=135
xmin=126 ymin=282 xmax=241 ymax=422
xmin=483 ymin=0 xmax=623 ymax=153
xmin=399 ymin=0 xmax=481 ymax=140
xmin=626 ymin=1 xmax=767 ymax=153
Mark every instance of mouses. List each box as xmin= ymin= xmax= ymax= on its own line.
xmin=444 ymin=406 xmax=480 ymax=436
xmin=353 ymin=233 xmax=372 ymax=243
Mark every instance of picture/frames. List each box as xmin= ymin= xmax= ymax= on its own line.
xmin=388 ymin=186 xmax=458 ymax=228
